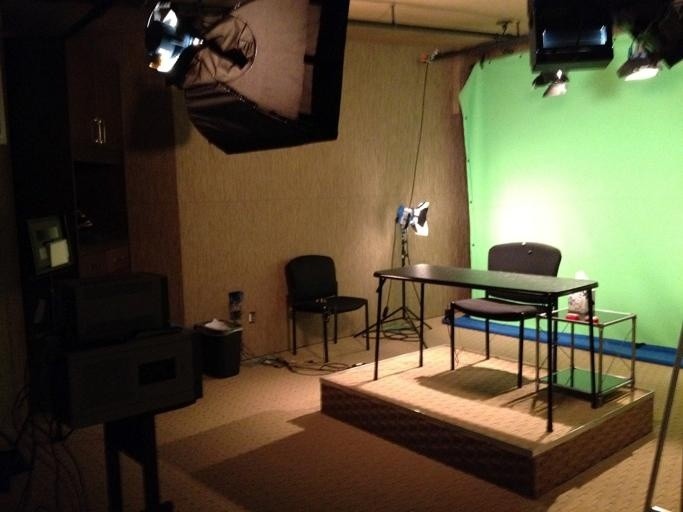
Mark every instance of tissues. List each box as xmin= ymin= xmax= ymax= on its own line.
xmin=567 ymin=269 xmax=597 ymax=316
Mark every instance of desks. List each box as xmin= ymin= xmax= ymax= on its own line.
xmin=373 ymin=263 xmax=597 ymax=432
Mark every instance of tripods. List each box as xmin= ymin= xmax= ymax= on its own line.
xmin=353 ymin=225 xmax=432 ymax=349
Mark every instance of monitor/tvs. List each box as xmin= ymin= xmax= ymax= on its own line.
xmin=181 ymin=1 xmax=350 ymax=155
xmin=527 ymin=1 xmax=614 ymax=71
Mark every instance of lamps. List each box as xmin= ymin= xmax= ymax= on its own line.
xmin=525 ymin=0 xmax=683 ymax=99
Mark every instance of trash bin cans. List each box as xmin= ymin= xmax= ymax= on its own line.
xmin=192 ymin=318 xmax=244 ymax=378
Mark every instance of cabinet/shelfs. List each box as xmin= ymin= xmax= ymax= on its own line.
xmin=534 ymin=307 xmax=637 ymax=409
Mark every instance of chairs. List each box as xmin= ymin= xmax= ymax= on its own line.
xmin=447 ymin=241 xmax=561 ymax=390
xmin=284 ymin=255 xmax=369 ymax=363
